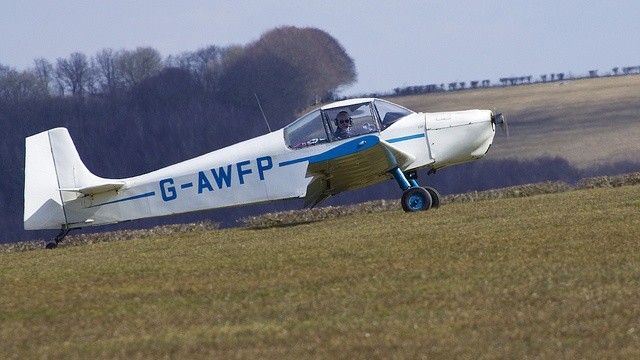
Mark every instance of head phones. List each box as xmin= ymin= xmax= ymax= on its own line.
xmin=334 ymin=111 xmax=353 ymax=126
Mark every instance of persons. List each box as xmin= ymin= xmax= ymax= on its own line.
xmin=334 ymin=111 xmax=356 ymax=139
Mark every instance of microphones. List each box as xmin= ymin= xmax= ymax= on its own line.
xmin=345 ymin=125 xmax=352 ymax=128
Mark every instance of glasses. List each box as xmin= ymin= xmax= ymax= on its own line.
xmin=339 ymin=119 xmax=350 ymax=124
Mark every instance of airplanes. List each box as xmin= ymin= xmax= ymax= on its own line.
xmin=23 ymin=89 xmax=510 ymax=249
